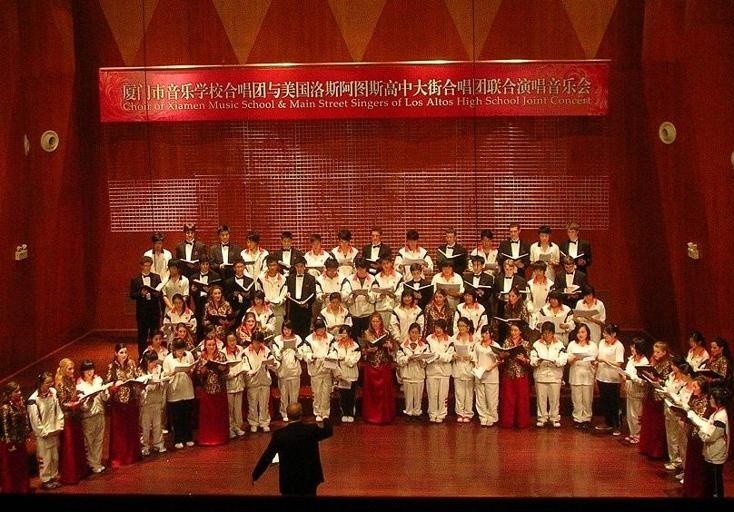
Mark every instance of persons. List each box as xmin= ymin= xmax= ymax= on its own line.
xmin=0 ymin=358 xmax=110 ymax=495
xmin=619 ymin=337 xmax=673 ymax=442
xmin=653 ymin=330 xmax=730 ymax=496
xmin=106 ymin=331 xmax=197 ymax=455
xmin=251 ymin=402 xmax=333 ymax=496
xmin=341 ymin=224 xmax=624 ymax=430
xmin=131 ymin=222 xmax=359 ymax=445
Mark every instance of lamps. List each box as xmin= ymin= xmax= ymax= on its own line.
xmin=12 ymin=242 xmax=29 ymax=260
xmin=685 ymin=240 xmax=698 ymax=261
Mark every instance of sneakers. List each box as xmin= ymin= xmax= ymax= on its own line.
xmin=229 ymin=423 xmax=271 ymax=436
xmin=143 ymin=441 xmax=194 ymax=456
xmin=536 ymin=420 xmax=639 ymax=443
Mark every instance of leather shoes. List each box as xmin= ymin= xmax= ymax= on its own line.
xmin=404 ymin=416 xmax=493 ymax=426
xmin=39 ymin=465 xmax=106 ymax=489
xmin=283 ymin=416 xmax=355 ymax=424
